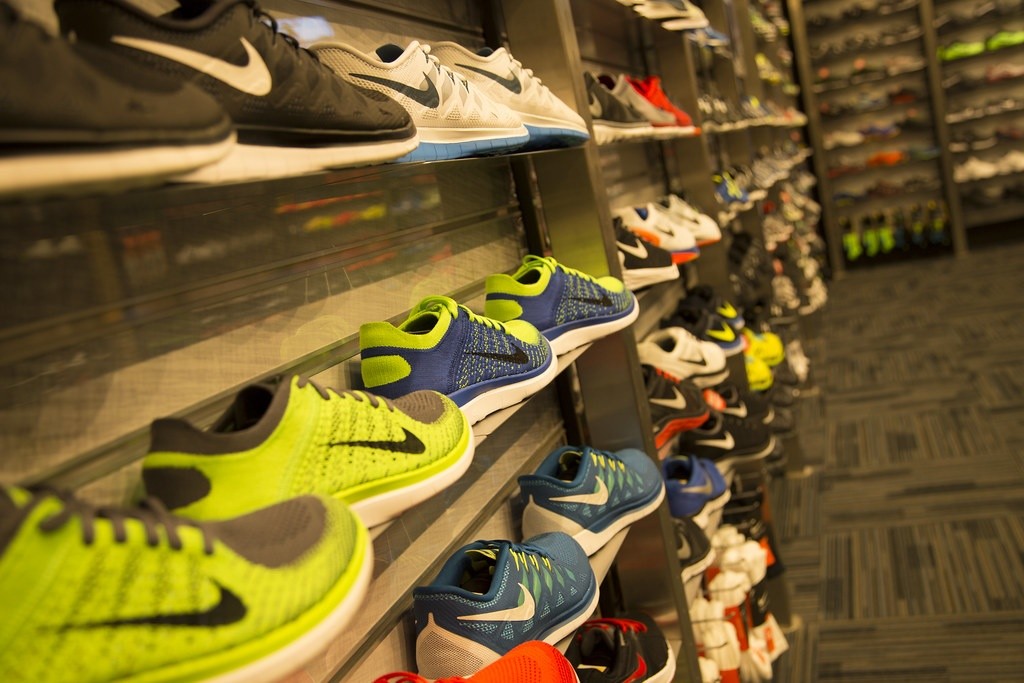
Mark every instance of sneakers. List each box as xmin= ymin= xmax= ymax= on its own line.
xmin=141 ymin=371 xmax=474 ymax=532
xmin=0 ymin=479 xmax=373 ymax=683
xmin=579 ymin=71 xmax=656 ymax=146
xmin=569 ymin=609 xmax=676 ymax=683
xmin=427 ymin=42 xmax=590 ymax=147
xmin=372 ymin=639 xmax=580 ymax=683
xmin=600 ymin=0 xmax=1024 ymax=683
xmin=53 ymin=0 xmax=419 ymax=185
xmin=0 ymin=0 xmax=238 ymax=198
xmin=360 ymin=294 xmax=557 ymax=427
xmin=485 ymin=256 xmax=640 ymax=358
xmin=307 ymin=39 xmax=530 ymax=165
xmin=517 ymin=444 xmax=665 ymax=557
xmin=411 ymin=530 xmax=600 ymax=681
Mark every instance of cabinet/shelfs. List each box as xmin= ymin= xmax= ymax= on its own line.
xmin=0 ymin=0 xmax=1024 ymax=683
